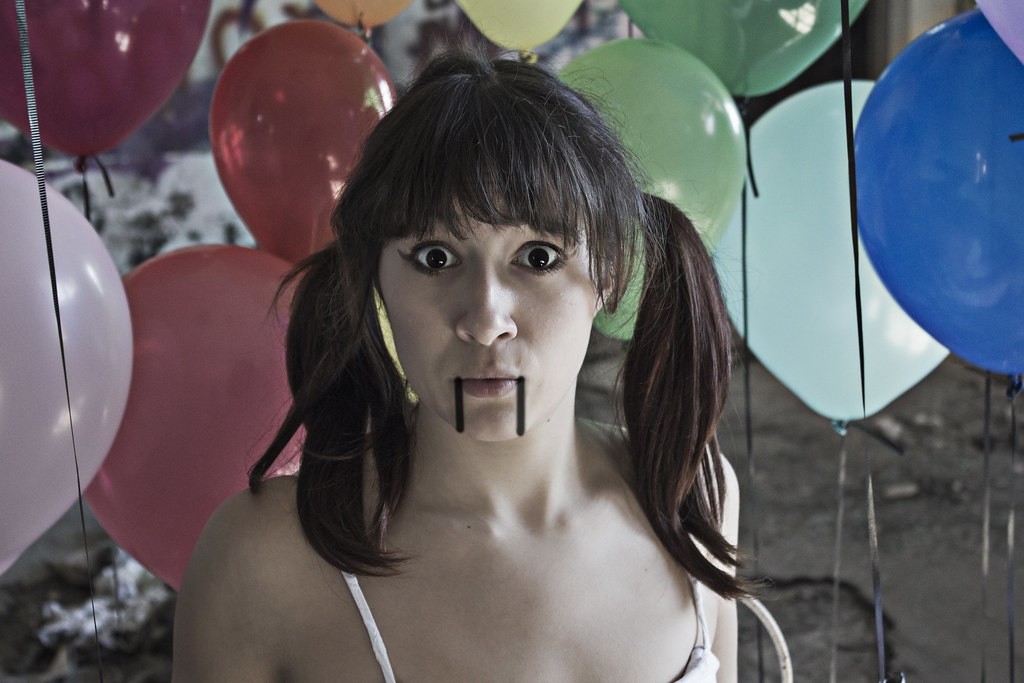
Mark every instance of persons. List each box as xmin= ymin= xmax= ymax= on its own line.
xmin=172 ymin=51 xmax=741 ymax=683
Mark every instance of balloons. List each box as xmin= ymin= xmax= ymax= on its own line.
xmin=0 ymin=0 xmax=1024 ymax=582
